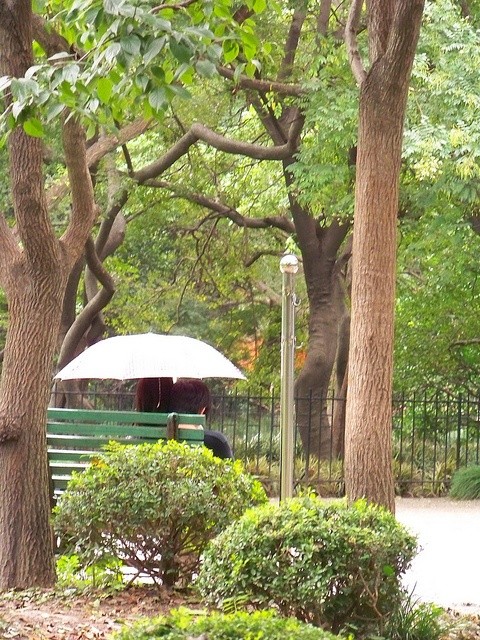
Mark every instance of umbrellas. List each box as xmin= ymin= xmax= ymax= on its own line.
xmin=52 ymin=332 xmax=249 ymax=382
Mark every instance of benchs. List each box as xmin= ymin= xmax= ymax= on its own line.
xmin=46 ymin=408 xmax=206 ymax=515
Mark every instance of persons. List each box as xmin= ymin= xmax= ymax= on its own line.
xmin=168 ymin=378 xmax=235 ymax=465
xmin=133 ymin=377 xmax=174 ymax=440
